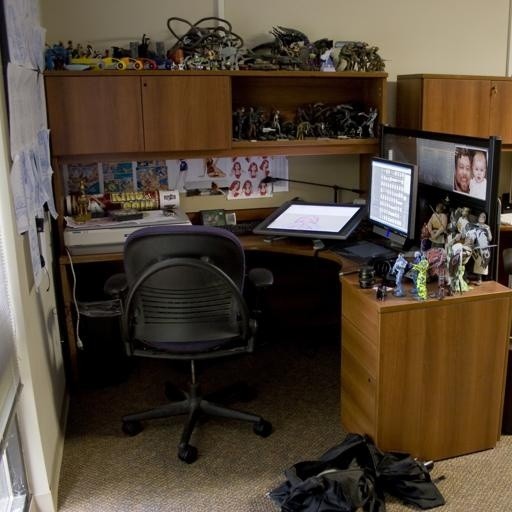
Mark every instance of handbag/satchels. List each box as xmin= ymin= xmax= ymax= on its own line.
xmin=266 ymin=432 xmax=386 ymax=512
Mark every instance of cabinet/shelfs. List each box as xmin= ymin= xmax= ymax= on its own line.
xmin=44 ymin=71 xmax=389 ymax=158
xmin=399 ymin=74 xmax=512 ymax=145
xmin=339 ymin=276 xmax=512 ymax=464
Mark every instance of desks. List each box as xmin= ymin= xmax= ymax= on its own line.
xmin=58 ymin=233 xmax=512 ymax=461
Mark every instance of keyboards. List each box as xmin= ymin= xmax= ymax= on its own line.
xmin=216 ymin=222 xmax=259 ymax=236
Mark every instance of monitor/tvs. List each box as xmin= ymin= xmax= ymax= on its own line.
xmin=366 ymin=156 xmax=418 ymax=247
xmin=253 ymin=200 xmax=367 ymax=249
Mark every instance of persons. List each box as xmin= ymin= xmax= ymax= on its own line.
xmin=233 ymin=102 xmax=378 ymax=139
xmin=198 ymin=158 xmax=227 ymax=178
xmin=44 ymin=41 xmax=92 ymax=71
xmin=469 ymin=152 xmax=487 ymax=200
xmin=175 ymin=160 xmax=188 ymax=189
xmin=208 ymin=182 xmax=218 ymax=192
xmin=289 ymin=42 xmax=383 ymax=72
xmin=229 ymin=155 xmax=269 ymax=197
xmin=455 ymin=148 xmax=471 ymax=193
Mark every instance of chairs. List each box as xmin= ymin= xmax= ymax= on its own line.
xmin=103 ymin=226 xmax=275 ymax=464
